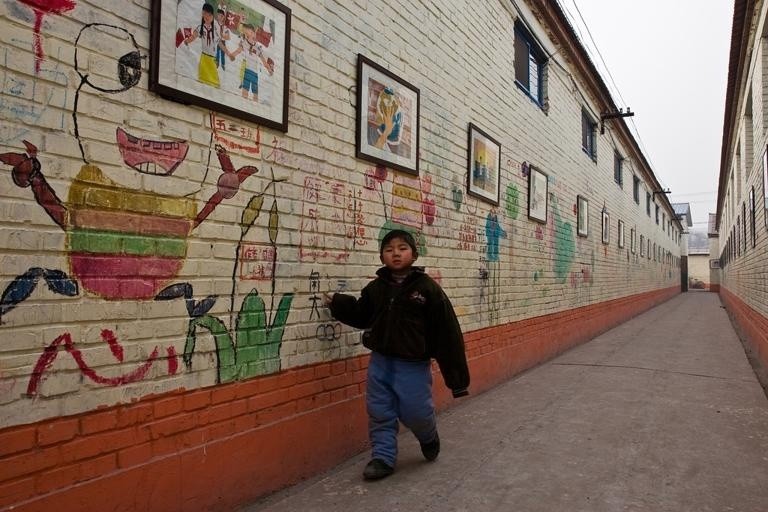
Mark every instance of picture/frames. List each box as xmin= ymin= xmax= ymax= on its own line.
xmin=577 ymin=195 xmax=676 ymax=267
xmin=149 ymin=0 xmax=291 ymax=131
xmin=356 ymin=54 xmax=420 ymax=175
xmin=467 ymin=122 xmax=501 ymax=206
xmin=528 ymin=165 xmax=548 ymax=224
xmin=710 ymin=148 xmax=768 ymax=269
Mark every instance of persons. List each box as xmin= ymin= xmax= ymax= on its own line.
xmin=323 ymin=229 xmax=470 ymax=478
xmin=183 ymin=2 xmax=274 ymax=102
xmin=374 ymin=106 xmax=395 ymax=150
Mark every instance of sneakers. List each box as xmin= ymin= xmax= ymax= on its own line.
xmin=364 ymin=459 xmax=394 ymax=479
xmin=422 ymin=432 xmax=440 ymax=461
